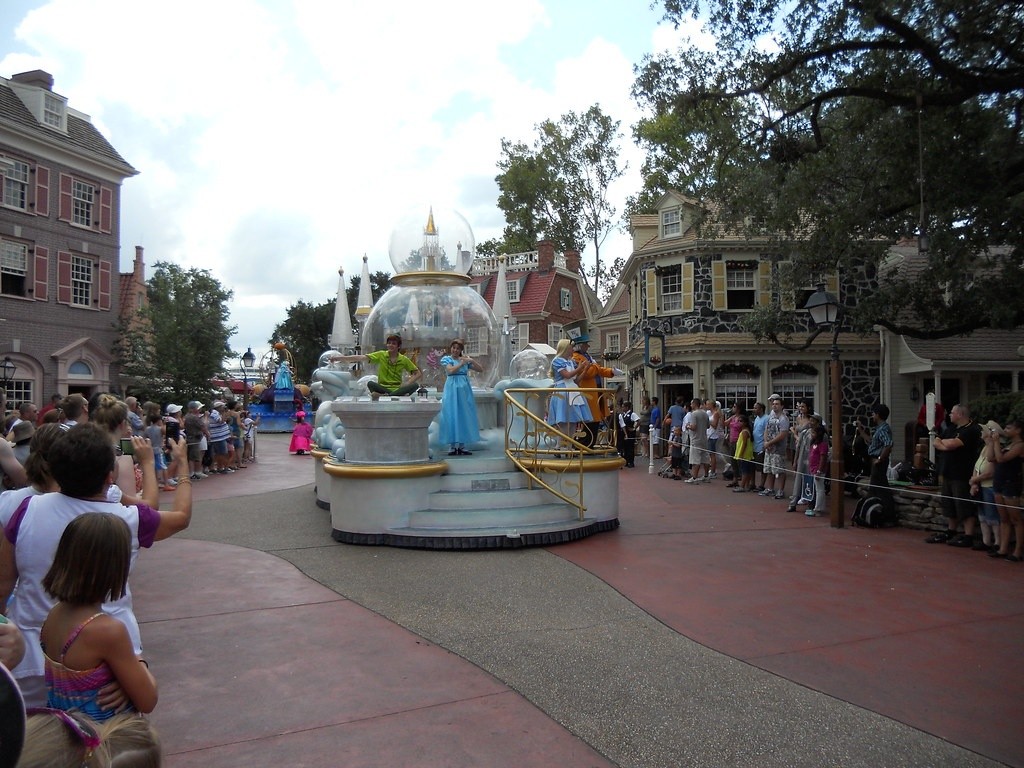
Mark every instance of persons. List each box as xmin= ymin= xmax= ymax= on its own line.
xmin=918 ymin=404 xmax=1024 ymax=562
xmin=276 ymin=361 xmax=294 ymax=390
xmin=0 ymin=387 xmax=260 ymax=493
xmin=0 ymin=423 xmax=160 ymax=615
xmin=439 ymin=340 xmax=483 ymax=455
xmin=328 ymin=335 xmax=422 ymax=396
xmin=39 ymin=513 xmax=158 ymax=725
xmin=0 ymin=423 xmax=192 ymax=707
xmin=548 ymin=339 xmax=595 ymax=458
xmin=605 ymin=393 xmax=829 ymax=516
xmin=856 ymin=404 xmax=895 ymax=527
xmin=289 ymin=405 xmax=314 ymax=454
xmin=572 ymin=342 xmax=622 ymax=456
xmin=0 ymin=613 xmax=161 ymax=768
xmin=266 ymin=357 xmax=279 ymax=383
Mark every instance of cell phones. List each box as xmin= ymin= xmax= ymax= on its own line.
xmin=165 ymin=422 xmax=180 ymax=449
xmin=121 ymin=438 xmax=135 ymax=456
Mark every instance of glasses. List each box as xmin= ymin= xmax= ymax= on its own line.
xmin=56 ymin=407 xmax=64 ymax=422
xmin=0 ymin=401 xmax=10 ymax=409
xmin=25 ymin=704 xmax=101 ymax=768
xmin=738 ymin=419 xmax=743 ymax=422
xmin=33 ymin=410 xmax=38 ymax=414
xmin=795 ymin=404 xmax=798 ymax=408
xmin=799 ymin=405 xmax=807 ymax=410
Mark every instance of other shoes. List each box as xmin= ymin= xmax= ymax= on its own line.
xmin=566 ymin=453 xmax=579 ymax=458
xmin=553 ymin=448 xmax=561 ymax=459
xmin=985 ymin=549 xmax=1009 ymax=559
xmin=1005 ymin=553 xmax=1024 ymax=562
xmin=971 ymin=538 xmax=1000 ymax=554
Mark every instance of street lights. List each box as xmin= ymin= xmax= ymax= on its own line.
xmin=237 ymin=344 xmax=256 ymax=410
xmin=801 ymin=281 xmax=846 ymax=528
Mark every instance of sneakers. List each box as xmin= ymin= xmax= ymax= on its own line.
xmin=669 ymin=468 xmax=823 ymax=517
xmin=925 ymin=530 xmax=957 ymax=544
xmin=157 ymin=456 xmax=254 ymax=491
xmin=946 ymin=533 xmax=973 ymax=548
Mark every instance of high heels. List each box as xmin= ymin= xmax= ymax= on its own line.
xmin=457 ymin=447 xmax=472 ymax=455
xmin=448 ymin=447 xmax=458 ymax=456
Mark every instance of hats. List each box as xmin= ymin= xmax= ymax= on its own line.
xmin=11 ymin=420 xmax=36 ymax=444
xmin=142 ymin=400 xmax=153 ymax=415
xmin=806 ymin=414 xmax=823 ymax=423
xmin=978 ymin=420 xmax=1005 ymax=436
xmin=767 ymin=394 xmax=780 ymax=400
xmin=213 ymin=401 xmax=227 ymax=408
xmin=561 ymin=317 xmax=594 ymax=346
xmin=166 ymin=403 xmax=184 ymax=414
xmin=193 ymin=400 xmax=206 ymax=411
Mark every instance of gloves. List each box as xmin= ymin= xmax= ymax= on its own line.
xmin=611 ymin=365 xmax=623 ymax=376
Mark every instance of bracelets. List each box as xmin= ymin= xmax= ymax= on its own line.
xmin=817 ymin=470 xmax=820 ymax=473
xmin=139 ymin=660 xmax=148 ymax=669
xmin=878 ymin=456 xmax=883 ymax=460
xmin=177 ymin=476 xmax=192 ymax=486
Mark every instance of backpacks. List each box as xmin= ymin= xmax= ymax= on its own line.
xmin=850 ymin=493 xmax=887 ymax=530
